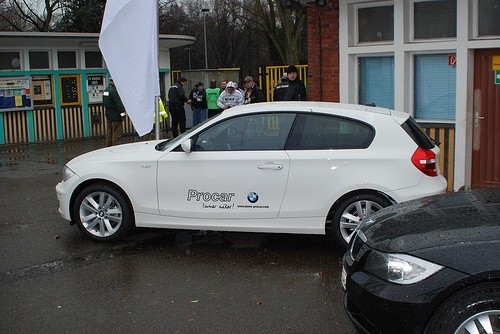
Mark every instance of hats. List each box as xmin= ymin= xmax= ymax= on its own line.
xmin=287 ymin=65 xmax=298 ymax=76
xmin=226 ymin=81 xmax=235 ymax=88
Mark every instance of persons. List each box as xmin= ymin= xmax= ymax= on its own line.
xmin=168 ymin=65 xmax=307 ymax=138
xmin=103 ymin=78 xmax=125 ymax=147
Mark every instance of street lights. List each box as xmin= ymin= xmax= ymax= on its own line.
xmin=201 ymin=8 xmax=210 ymax=89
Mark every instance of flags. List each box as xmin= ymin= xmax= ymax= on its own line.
xmin=97 ymin=0 xmax=161 ymax=137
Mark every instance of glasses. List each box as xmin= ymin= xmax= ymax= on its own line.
xmin=244 ymin=80 xmax=252 ymax=83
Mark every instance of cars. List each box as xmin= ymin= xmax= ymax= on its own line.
xmin=341 ymin=186 xmax=500 ymax=334
xmin=55 ymin=101 xmax=448 ymax=252
